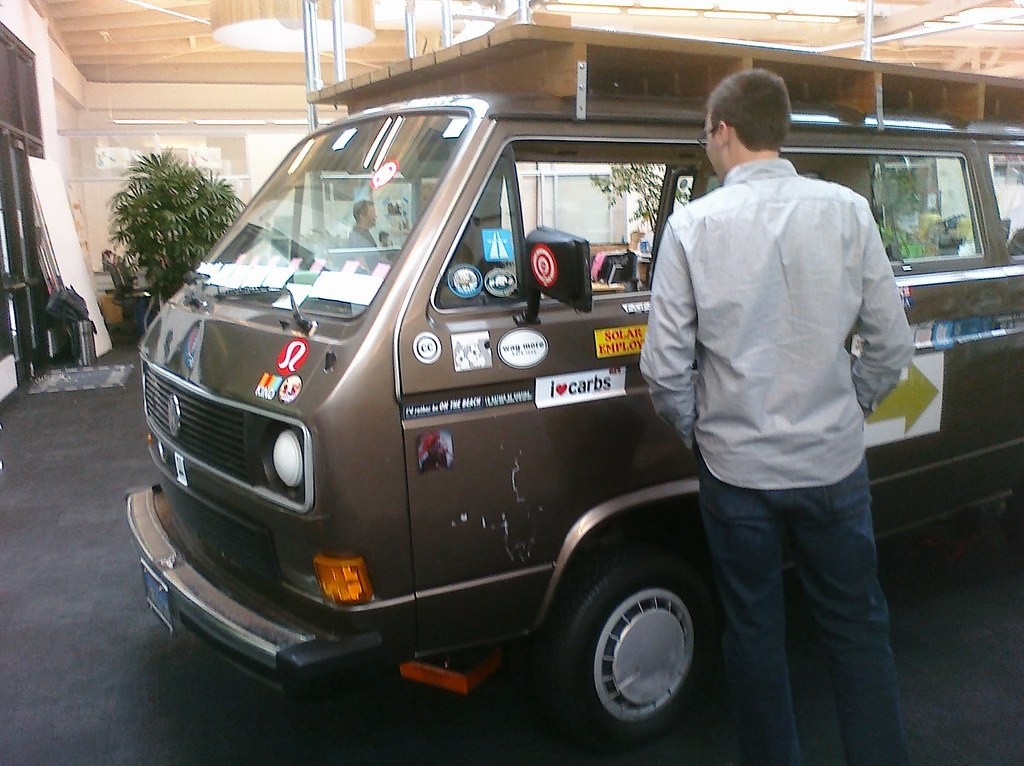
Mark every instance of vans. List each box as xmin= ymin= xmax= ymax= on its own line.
xmin=125 ymin=89 xmax=1024 ymax=741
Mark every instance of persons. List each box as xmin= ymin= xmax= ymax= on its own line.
xmin=637 ymin=68 xmax=919 ymax=765
xmin=377 ymin=232 xmax=391 ymax=247
xmin=347 ymin=199 xmax=380 ymax=269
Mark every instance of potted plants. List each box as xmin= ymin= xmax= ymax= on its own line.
xmin=104 ymin=148 xmax=248 ymax=313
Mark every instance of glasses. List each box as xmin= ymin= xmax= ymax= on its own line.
xmin=696 ymin=121 xmax=728 ymax=149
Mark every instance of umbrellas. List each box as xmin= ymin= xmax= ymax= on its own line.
xmin=43 ymin=272 xmax=101 ymax=338
